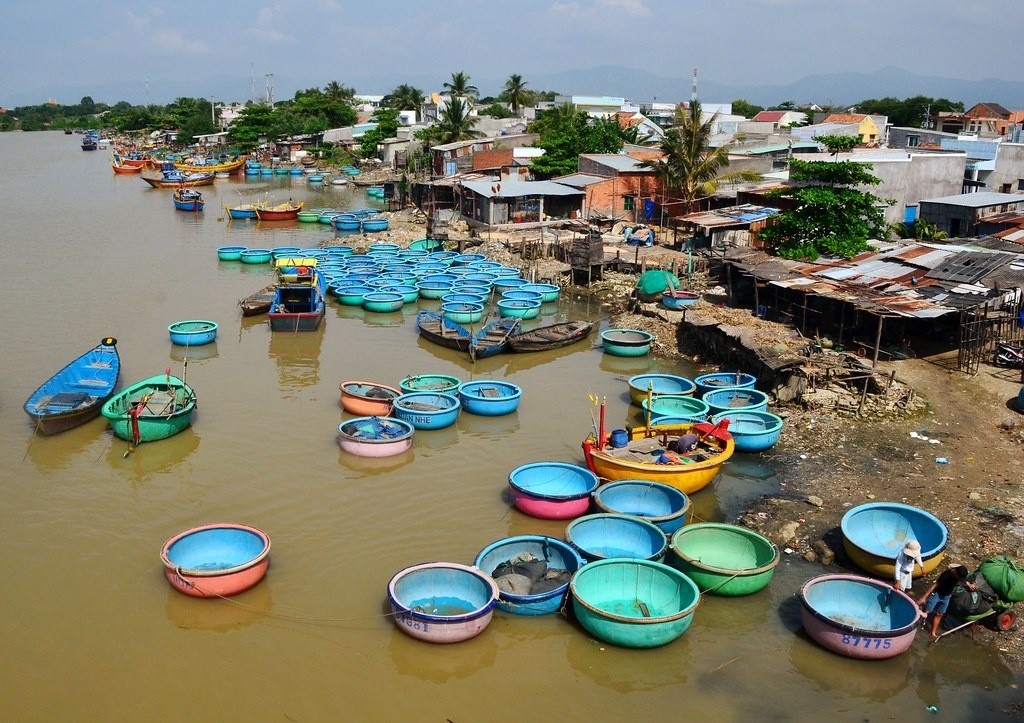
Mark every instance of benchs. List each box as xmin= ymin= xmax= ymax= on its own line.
xmin=95 ymin=349 xmax=116 ymax=353
xmin=131 ymin=396 xmax=179 ymax=404
xmin=63 ymin=382 xmax=112 ymax=390
xmin=82 ymin=366 xmax=117 ymax=371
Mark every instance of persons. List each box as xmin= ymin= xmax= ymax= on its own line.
xmin=893 ymin=541 xmax=926 ymax=593
xmin=916 ymin=566 xmax=976 ymax=637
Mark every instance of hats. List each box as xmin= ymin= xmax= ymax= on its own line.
xmin=903 ymin=540 xmax=921 ymax=557
xmin=956 ymin=566 xmax=968 ymax=582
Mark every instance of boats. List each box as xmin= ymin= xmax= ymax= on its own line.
xmin=582 ymin=381 xmax=734 ymax=496
xmin=101 ymin=358 xmax=196 ymax=459
xmin=254 ymin=190 xmax=304 ymax=221
xmin=469 ymin=316 xmax=522 ymax=360
xmin=505 ymin=319 xmax=594 ymax=354
xmin=222 ymin=188 xmax=268 ymax=221
xmin=65 ymin=129 xmax=247 ymax=188
xmin=173 ymin=175 xmax=205 ymax=211
xmin=236 ymin=257 xmax=326 ymax=332
xmin=23 ymin=336 xmax=120 ymax=437
xmin=415 ymin=307 xmax=472 ymax=352
